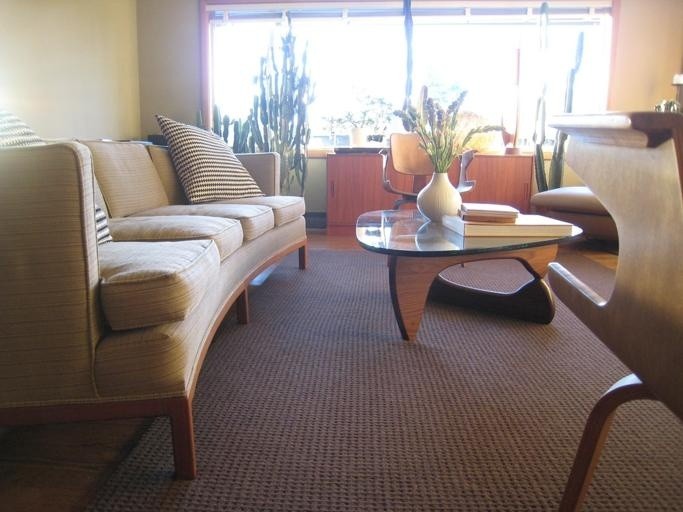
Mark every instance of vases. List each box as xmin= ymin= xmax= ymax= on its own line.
xmin=417 ymin=173 xmax=462 ymax=222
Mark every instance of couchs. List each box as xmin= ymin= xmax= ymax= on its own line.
xmin=4 ymin=138 xmax=308 ymax=481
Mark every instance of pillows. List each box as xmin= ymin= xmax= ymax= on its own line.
xmin=1 ymin=111 xmax=112 ymax=245
xmin=155 ymin=114 xmax=266 ymax=203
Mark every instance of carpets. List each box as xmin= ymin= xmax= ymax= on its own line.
xmin=84 ymin=246 xmax=683 ymax=512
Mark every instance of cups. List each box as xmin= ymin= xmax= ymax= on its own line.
xmin=653 ymin=99 xmax=681 ymax=114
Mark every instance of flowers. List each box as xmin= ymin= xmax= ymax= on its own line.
xmin=393 ymin=85 xmax=506 ymax=172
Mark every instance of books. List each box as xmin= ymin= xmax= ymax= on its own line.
xmin=441 ymin=202 xmax=573 ymax=238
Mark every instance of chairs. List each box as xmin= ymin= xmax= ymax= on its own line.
xmin=378 ymin=132 xmax=477 ymax=267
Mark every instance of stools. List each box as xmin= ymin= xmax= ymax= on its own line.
xmin=530 ymin=185 xmax=619 ymax=246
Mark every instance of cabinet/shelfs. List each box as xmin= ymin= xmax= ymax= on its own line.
xmin=326 ymin=152 xmax=536 ymax=236
